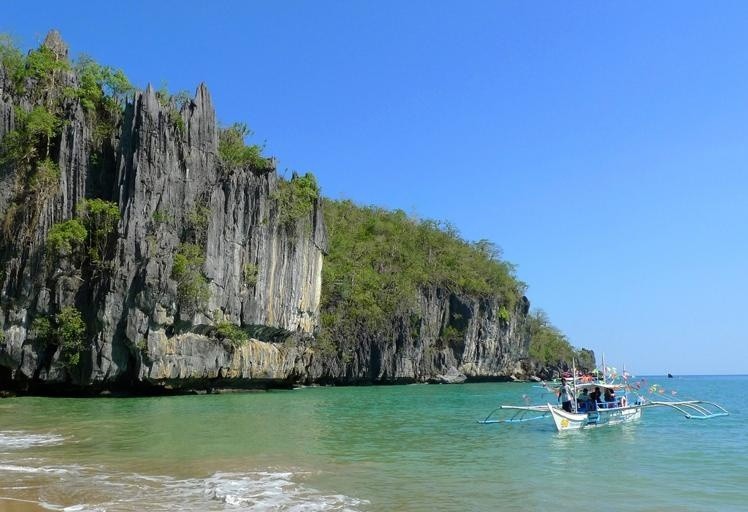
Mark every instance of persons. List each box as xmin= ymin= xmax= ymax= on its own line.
xmin=579 ymin=388 xmax=592 ymax=401
xmin=557 ymin=378 xmax=575 ymax=413
xmin=608 ymin=388 xmax=616 ymax=406
xmin=589 ymin=387 xmax=603 ymax=408
xmin=604 ymin=389 xmax=612 ymax=402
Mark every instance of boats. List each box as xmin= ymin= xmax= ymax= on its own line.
xmin=477 ymin=353 xmax=729 ymax=431
